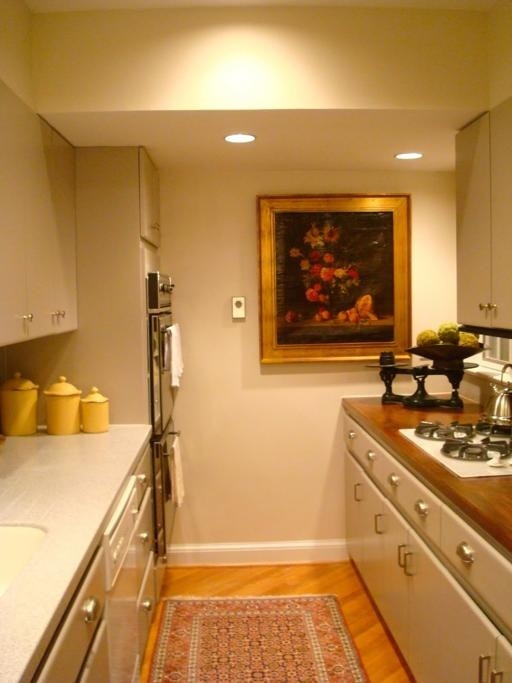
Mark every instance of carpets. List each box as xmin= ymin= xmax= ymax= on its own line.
xmin=146 ymin=589 xmax=369 ymax=683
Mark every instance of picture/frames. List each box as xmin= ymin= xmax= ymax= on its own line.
xmin=254 ymin=190 xmax=413 ymax=368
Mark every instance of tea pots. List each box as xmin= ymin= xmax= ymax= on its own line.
xmin=482 ymin=360 xmax=512 ymax=427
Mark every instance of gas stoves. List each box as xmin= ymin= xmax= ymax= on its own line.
xmin=396 ymin=416 xmax=512 ymax=479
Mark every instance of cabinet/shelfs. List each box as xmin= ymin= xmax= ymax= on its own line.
xmin=455 ymin=100 xmax=511 ymax=331
xmin=32 ymin=545 xmax=109 ymax=682
xmin=1 ymin=79 xmax=34 ymax=347
xmin=133 ymin=444 xmax=160 ymax=658
xmin=340 ymin=413 xmax=363 ymax=590
xmin=365 ymin=433 xmax=384 ymax=619
xmin=440 ymin=502 xmax=511 ymax=683
xmin=75 ymin=146 xmax=164 ymax=252
xmin=384 ymin=453 xmax=441 ymax=682
xmin=34 ymin=111 xmax=78 ymax=341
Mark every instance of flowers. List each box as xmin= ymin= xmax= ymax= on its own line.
xmin=284 ymin=220 xmax=361 ymax=324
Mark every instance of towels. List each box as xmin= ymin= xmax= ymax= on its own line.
xmin=165 ymin=321 xmax=186 ymax=394
xmin=170 ymin=438 xmax=187 ymax=513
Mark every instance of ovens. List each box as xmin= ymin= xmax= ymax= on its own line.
xmin=146 ymin=268 xmax=187 ymax=567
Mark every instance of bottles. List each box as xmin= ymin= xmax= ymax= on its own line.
xmin=1 ymin=370 xmax=111 ymax=435
